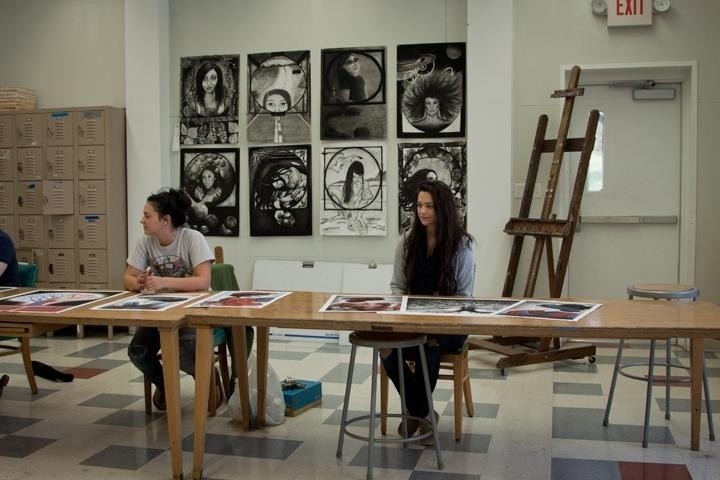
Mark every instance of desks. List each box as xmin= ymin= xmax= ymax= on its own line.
xmin=1 ymin=285 xmax=223 ymax=479
xmin=186 ymin=290 xmax=720 ymax=480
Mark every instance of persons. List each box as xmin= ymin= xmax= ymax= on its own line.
xmin=123 ymin=187 xmax=224 ymax=413
xmin=193 ymin=164 xmax=223 ymax=203
xmin=339 ymin=160 xmax=371 ymax=207
xmin=336 ymin=50 xmax=365 ymax=103
xmin=262 ymin=89 xmax=292 ymax=115
xmin=189 ymin=60 xmax=230 ymax=118
xmin=379 ymin=180 xmax=476 ymax=445
xmin=0 ymin=230 xmax=21 ymax=401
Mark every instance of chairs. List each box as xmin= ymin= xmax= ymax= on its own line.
xmin=0 ymin=259 xmax=42 ymax=397
xmin=139 ymin=244 xmax=234 ymax=418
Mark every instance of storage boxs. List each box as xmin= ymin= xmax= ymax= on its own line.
xmin=279 ymin=379 xmax=326 ymax=417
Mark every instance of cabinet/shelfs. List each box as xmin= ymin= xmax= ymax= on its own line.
xmin=0 ymin=105 xmax=127 ymax=291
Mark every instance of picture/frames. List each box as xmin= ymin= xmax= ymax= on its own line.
xmin=397 ymin=141 xmax=467 ymax=239
xmin=180 ymin=147 xmax=240 ymax=237
xmin=250 ymin=143 xmax=313 ymax=237
xmin=396 ymin=42 xmax=466 ymax=139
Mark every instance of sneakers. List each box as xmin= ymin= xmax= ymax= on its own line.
xmin=397 ymin=411 xmax=420 ymax=440
xmin=419 ymin=409 xmax=442 ymax=447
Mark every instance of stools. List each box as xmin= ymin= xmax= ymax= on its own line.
xmin=332 ymin=329 xmax=446 ymax=480
xmin=378 ymin=339 xmax=477 ymax=442
xmin=602 ymin=282 xmax=716 ymax=452
